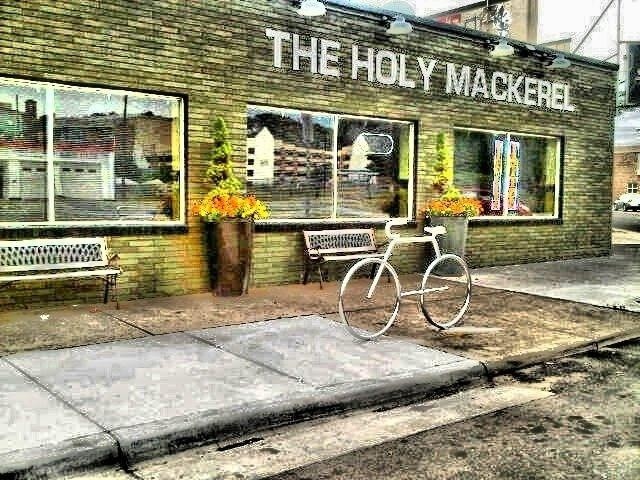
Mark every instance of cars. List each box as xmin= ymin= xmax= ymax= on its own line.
xmin=613 ymin=194 xmax=640 ymax=211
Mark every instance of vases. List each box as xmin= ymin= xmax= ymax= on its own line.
xmin=429 ymin=216 xmax=468 ymax=278
xmin=204 ymin=217 xmax=253 ymax=297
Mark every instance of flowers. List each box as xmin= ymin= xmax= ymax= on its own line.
xmin=422 ymin=189 xmax=485 ymax=217
xmin=193 ymin=186 xmax=273 ymax=224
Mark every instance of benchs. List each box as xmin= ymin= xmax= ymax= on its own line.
xmin=0 ymin=237 xmax=122 ymax=311
xmin=301 ymin=226 xmax=394 ymax=289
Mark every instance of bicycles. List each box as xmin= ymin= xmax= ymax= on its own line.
xmin=339 ymin=218 xmax=471 ymax=340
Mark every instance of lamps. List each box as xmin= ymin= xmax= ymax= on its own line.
xmin=386 ymin=14 xmax=413 ymax=35
xmin=295 ymin=0 xmax=326 ymax=17
xmin=489 ymin=35 xmax=515 ymax=57
